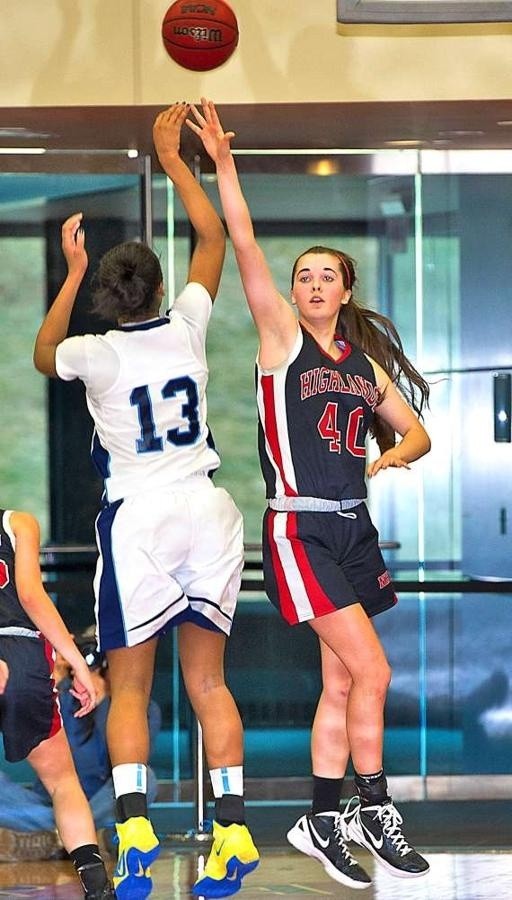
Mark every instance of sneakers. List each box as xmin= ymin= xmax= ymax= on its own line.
xmin=112 ymin=816 xmax=162 ymax=900
xmin=285 ymin=813 xmax=373 ymax=890
xmin=190 ymin=819 xmax=259 ymax=900
xmin=72 ymin=856 xmax=115 ymax=900
xmin=346 ymin=800 xmax=430 ymax=879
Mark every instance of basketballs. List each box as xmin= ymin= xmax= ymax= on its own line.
xmin=162 ymin=1 xmax=238 ymax=70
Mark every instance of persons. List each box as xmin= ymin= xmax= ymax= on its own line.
xmin=29 ymin=101 xmax=266 ymax=899
xmin=2 ymin=618 xmax=164 ymax=864
xmin=177 ymin=92 xmax=435 ymax=892
xmin=0 ymin=507 xmax=116 ymax=900
